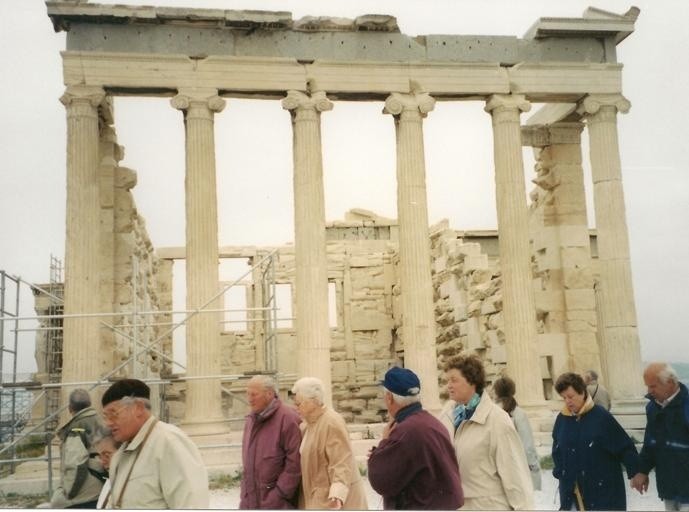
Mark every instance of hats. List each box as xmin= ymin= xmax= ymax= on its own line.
xmin=378 ymin=366 xmax=420 ymax=395
xmin=102 ymin=379 xmax=151 ymax=407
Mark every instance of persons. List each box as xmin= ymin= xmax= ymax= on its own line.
xmin=239 ymin=375 xmax=304 ymax=510
xmin=49 ymin=388 xmax=101 ymax=509
xmin=102 ymin=379 xmax=210 ymax=510
xmin=95 ymin=431 xmax=123 ymax=510
xmin=434 ymin=357 xmax=534 ymax=511
xmin=551 ymin=373 xmax=639 ymax=511
xmin=583 ymin=371 xmax=612 ymax=414
xmin=291 ymin=378 xmax=367 ymax=510
xmin=634 ymin=362 xmax=689 ymax=512
xmin=366 ymin=366 xmax=465 ymax=511
xmin=492 ymin=378 xmax=542 ymax=491
